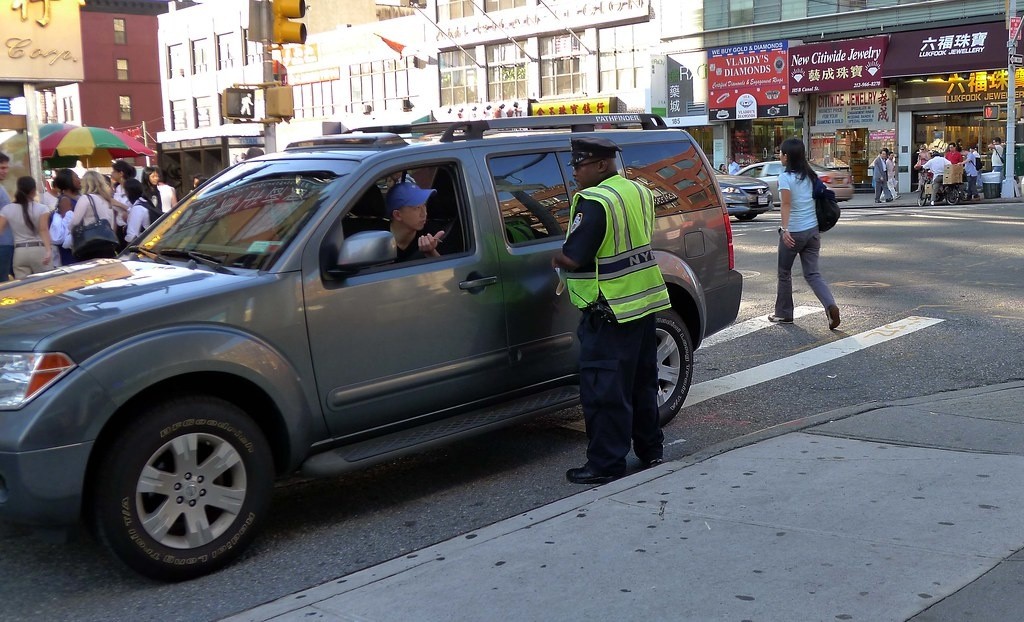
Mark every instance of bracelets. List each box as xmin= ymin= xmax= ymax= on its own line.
xmin=781 ymin=227 xmax=788 ymax=232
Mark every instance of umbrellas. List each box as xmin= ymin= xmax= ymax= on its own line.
xmin=39 ymin=127 xmax=156 ymax=171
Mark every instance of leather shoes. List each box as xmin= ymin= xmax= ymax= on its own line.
xmin=567 ymin=465 xmax=615 ymax=484
xmin=640 ymin=454 xmax=662 ymax=467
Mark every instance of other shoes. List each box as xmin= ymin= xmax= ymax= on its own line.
xmin=887 ymin=197 xmax=893 ymax=202
xmin=973 ymin=197 xmax=981 ymax=201
xmin=963 ymin=197 xmax=970 ymax=200
xmin=931 ymin=201 xmax=935 ymax=206
xmin=768 ymin=312 xmax=793 ymax=324
xmin=875 ymin=199 xmax=882 ymax=203
xmin=827 ymin=305 xmax=840 ymax=330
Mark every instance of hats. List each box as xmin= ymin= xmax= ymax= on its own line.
xmin=566 ymin=135 xmax=622 ymax=166
xmin=385 ymin=184 xmax=436 ymax=216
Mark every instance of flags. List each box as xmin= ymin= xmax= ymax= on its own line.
xmin=381 ymin=37 xmax=405 ymax=60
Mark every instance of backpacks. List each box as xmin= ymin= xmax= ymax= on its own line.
xmin=968 ymin=154 xmax=982 ymax=171
xmin=138 ymin=198 xmax=164 ymax=225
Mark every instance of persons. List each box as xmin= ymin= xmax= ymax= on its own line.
xmin=768 ymin=138 xmax=840 ymax=330
xmin=915 ymin=143 xmax=980 ymax=207
xmin=385 ymin=182 xmax=444 ymax=264
xmin=728 ymin=156 xmax=740 ymax=175
xmin=988 ymin=137 xmax=1003 ymax=172
xmin=551 ymin=136 xmax=672 ymax=483
xmin=719 ymin=164 xmax=726 ymax=172
xmin=456 ymin=218 xmax=551 ymax=389
xmin=873 ymin=149 xmax=901 ymax=204
xmin=0 ymin=152 xmax=178 ymax=285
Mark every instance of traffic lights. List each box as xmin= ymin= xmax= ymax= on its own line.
xmin=273 ymin=0 xmax=307 ymax=44
xmin=222 ymin=88 xmax=255 ymax=120
xmin=266 ymin=85 xmax=294 ymax=120
xmin=981 ymin=104 xmax=1001 ymax=122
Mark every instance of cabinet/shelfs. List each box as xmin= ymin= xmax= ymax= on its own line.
xmin=836 ymin=129 xmax=865 ymax=159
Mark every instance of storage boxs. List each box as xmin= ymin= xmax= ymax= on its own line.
xmin=925 ymin=183 xmax=941 ymax=194
xmin=944 ymin=164 xmax=963 ymax=184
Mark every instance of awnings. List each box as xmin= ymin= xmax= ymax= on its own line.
xmin=881 ymin=21 xmax=1024 ymax=78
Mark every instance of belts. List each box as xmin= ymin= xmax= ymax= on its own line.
xmin=15 ymin=242 xmax=44 ymax=247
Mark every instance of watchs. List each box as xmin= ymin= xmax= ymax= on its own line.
xmin=774 ymin=56 xmax=784 ymax=75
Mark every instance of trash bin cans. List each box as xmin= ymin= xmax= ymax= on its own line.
xmin=981 ymin=172 xmax=1000 ymax=198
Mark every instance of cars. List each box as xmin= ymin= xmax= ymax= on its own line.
xmin=712 ymin=168 xmax=774 ymax=221
xmin=734 ymin=160 xmax=854 ymax=203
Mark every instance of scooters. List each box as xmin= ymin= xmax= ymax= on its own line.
xmin=917 ymin=170 xmax=962 ymax=204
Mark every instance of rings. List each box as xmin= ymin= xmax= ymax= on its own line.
xmin=716 ymin=110 xmax=729 ymax=119
xmin=765 ymin=90 xmax=780 ymax=99
xmin=767 ymin=107 xmax=780 ymax=115
xmin=717 ymin=93 xmax=729 ymax=104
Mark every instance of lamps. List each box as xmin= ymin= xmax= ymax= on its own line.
xmin=821 ymin=32 xmax=824 ymax=38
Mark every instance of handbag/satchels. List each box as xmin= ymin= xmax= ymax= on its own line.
xmin=71 ymin=193 xmax=120 ymax=257
xmin=1001 ymin=155 xmax=1005 ymax=164
xmin=811 ymin=173 xmax=840 ymax=232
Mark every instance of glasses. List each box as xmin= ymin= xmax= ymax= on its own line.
xmin=573 ymin=158 xmax=607 ymax=171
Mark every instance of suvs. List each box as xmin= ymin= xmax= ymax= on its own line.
xmin=0 ymin=112 xmax=749 ymax=585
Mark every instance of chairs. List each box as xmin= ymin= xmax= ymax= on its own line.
xmin=344 ymin=166 xmax=464 ymax=261
